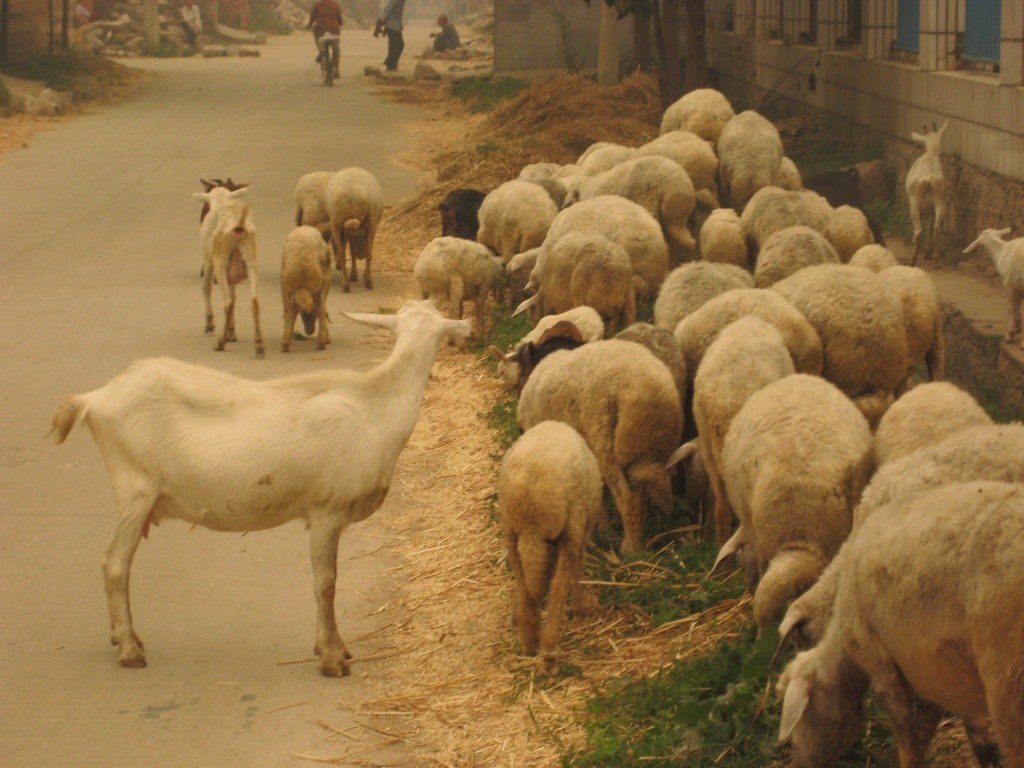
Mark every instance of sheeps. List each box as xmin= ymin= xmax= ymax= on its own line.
xmin=281 ymin=225 xmax=332 ymax=354
xmin=705 ymin=372 xmax=1023 ymax=768
xmin=293 ymin=167 xmax=383 ymax=292
xmin=963 ymin=227 xmax=1023 ymax=342
xmin=414 ymin=227 xmax=946 ymax=555
xmin=905 ymin=121 xmax=949 ymax=265
xmin=437 ymin=88 xmax=884 ymax=265
xmin=497 ymin=419 xmax=603 ymax=681
xmin=46 ymin=298 xmax=473 ymax=678
xmin=192 ymin=178 xmax=266 ymax=360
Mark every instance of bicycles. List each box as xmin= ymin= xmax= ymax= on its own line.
xmin=319 ymin=36 xmax=339 ymax=87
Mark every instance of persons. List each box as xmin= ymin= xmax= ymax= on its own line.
xmin=180 ymin=0 xmax=202 ymax=46
xmin=430 ymin=16 xmax=458 ymax=52
xmin=305 ymin=0 xmax=342 ymax=81
xmin=74 ymin=0 xmax=130 ymax=47
xmin=374 ymin=0 xmax=405 ymax=71
